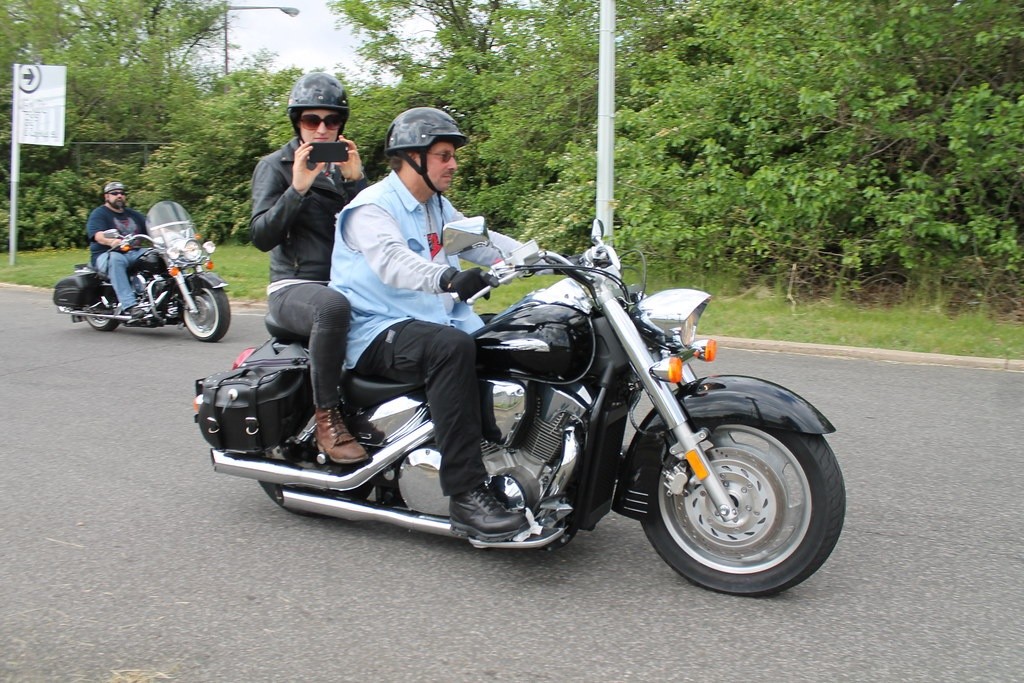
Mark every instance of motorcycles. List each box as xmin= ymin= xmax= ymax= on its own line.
xmin=191 ymin=220 xmax=850 ymax=599
xmin=52 ymin=200 xmax=232 ymax=344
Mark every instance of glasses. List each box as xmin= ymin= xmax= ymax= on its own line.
xmin=104 ymin=192 xmax=125 ymax=195
xmin=413 ymin=150 xmax=456 ymax=162
xmin=295 ymin=114 xmax=346 ymax=130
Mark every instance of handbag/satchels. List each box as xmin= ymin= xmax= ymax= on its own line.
xmin=197 ymin=336 xmax=311 ymax=453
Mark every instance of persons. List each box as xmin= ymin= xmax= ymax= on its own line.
xmin=327 ymin=106 xmax=587 ymax=542
xmin=250 ymin=73 xmax=368 ymax=464
xmin=85 ymin=182 xmax=174 ymax=319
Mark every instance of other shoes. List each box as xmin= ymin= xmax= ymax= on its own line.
xmin=127 ymin=305 xmax=145 ymax=319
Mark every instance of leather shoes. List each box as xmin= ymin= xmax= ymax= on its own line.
xmin=449 ymin=481 xmax=528 ymax=543
xmin=316 ymin=408 xmax=368 ymax=464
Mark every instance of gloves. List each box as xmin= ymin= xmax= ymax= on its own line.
xmin=553 ymin=254 xmax=589 ymax=275
xmin=439 ymin=266 xmax=500 ymax=305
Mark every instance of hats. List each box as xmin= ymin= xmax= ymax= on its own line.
xmin=103 ymin=181 xmax=124 ymax=194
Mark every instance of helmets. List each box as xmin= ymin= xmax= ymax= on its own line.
xmin=384 ymin=107 xmax=470 ymax=153
xmin=287 ymin=71 xmax=349 ymax=117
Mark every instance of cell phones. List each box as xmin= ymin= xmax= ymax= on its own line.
xmin=308 ymin=142 xmax=348 ymax=162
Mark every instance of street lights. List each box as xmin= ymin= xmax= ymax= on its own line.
xmin=223 ymin=4 xmax=301 ymax=94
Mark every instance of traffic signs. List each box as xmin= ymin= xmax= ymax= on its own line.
xmin=15 ymin=65 xmax=68 ymax=148
